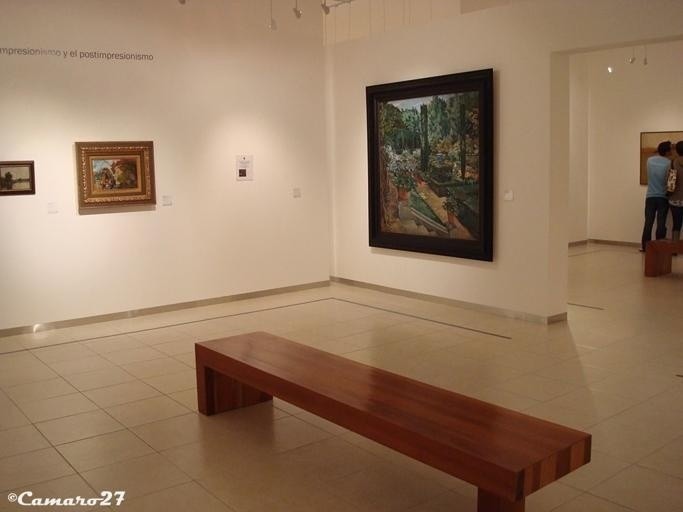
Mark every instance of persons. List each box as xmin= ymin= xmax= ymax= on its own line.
xmin=667 ymin=141 xmax=683 ymax=256
xmin=637 ymin=141 xmax=673 ymax=253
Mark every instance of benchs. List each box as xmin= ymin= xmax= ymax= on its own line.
xmin=644 ymin=239 xmax=682 ymax=276
xmin=194 ymin=331 xmax=591 ymax=512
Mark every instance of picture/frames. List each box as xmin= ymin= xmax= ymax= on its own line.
xmin=365 ymin=69 xmax=494 ymax=263
xmin=0 ymin=161 xmax=35 ymax=195
xmin=75 ymin=142 xmax=156 ymax=215
xmin=640 ymin=132 xmax=683 ymax=185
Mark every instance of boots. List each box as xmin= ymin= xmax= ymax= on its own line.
xmin=672 ymin=230 xmax=680 ymax=256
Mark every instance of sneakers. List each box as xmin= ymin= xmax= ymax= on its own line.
xmin=640 ymin=248 xmax=645 ymax=252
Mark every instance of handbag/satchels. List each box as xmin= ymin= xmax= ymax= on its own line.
xmin=666 ymin=159 xmax=678 ymax=192
